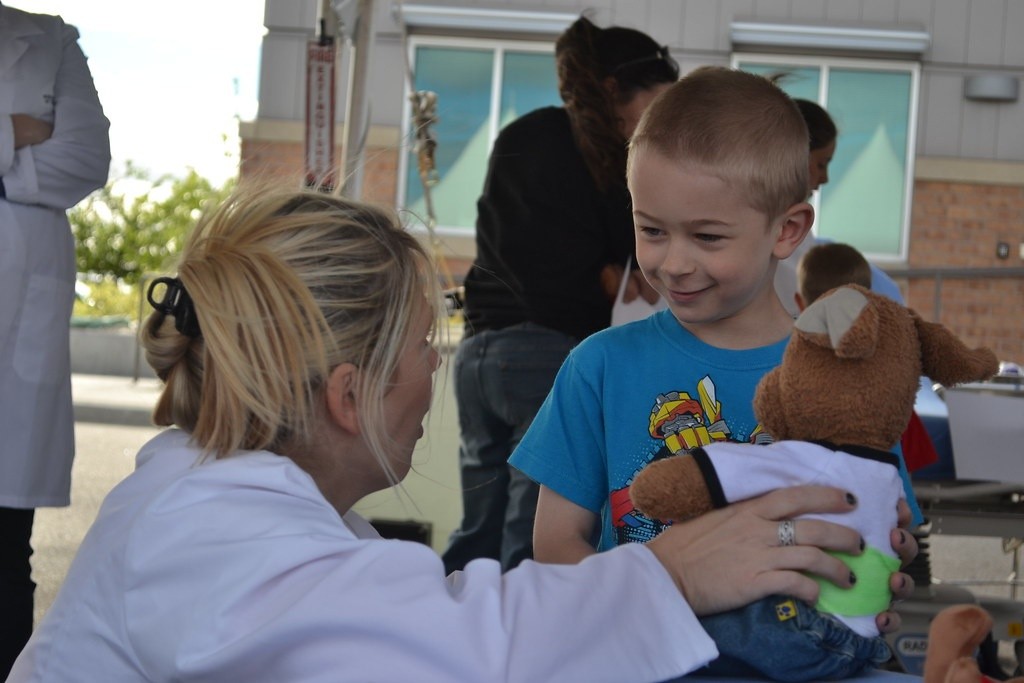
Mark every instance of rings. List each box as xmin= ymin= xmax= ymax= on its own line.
xmin=779 ymin=520 xmax=796 ymax=547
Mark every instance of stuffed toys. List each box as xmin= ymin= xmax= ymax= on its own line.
xmin=630 ymin=283 xmax=999 ymax=683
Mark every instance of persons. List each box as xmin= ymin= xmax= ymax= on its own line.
xmin=507 ymin=65 xmax=925 ymax=565
xmin=774 ymin=98 xmax=873 ymax=320
xmin=442 ymin=14 xmax=679 ymax=578
xmin=0 ymin=182 xmax=918 ymax=683
xmin=0 ymin=0 xmax=112 ymax=683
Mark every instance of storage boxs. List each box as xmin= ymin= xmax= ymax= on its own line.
xmin=934 ymin=383 xmax=1023 ymax=485
